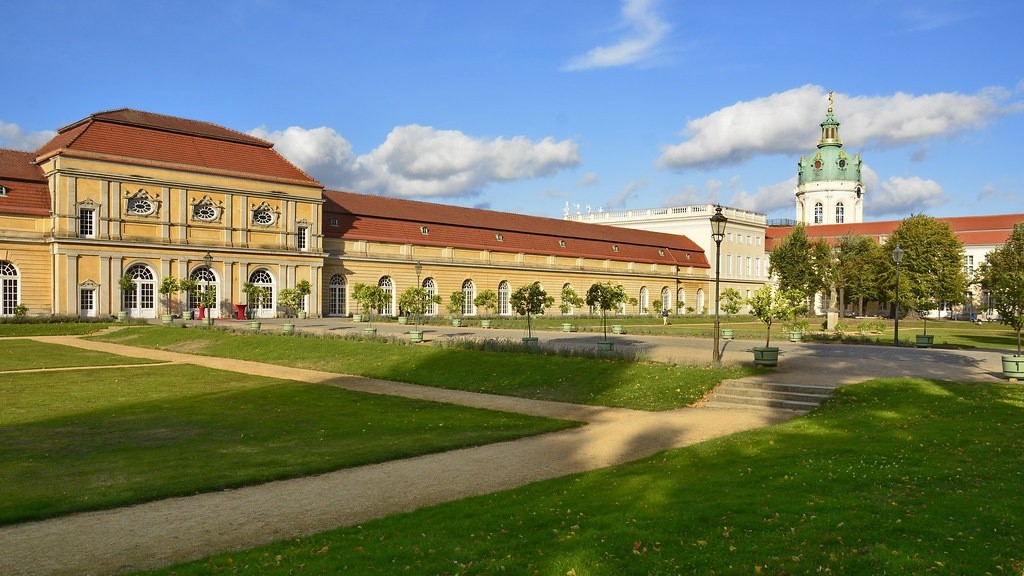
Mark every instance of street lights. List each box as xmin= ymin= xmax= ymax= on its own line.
xmin=892 ymin=244 xmax=904 ymax=346
xmin=414 ymin=260 xmax=423 ymax=325
xmin=203 ymin=250 xmax=214 ymax=325
xmin=708 ymin=202 xmax=728 ymax=368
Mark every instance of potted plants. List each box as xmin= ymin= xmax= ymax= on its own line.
xmin=399 ymin=287 xmax=443 ymax=343
xmin=158 ymin=276 xmax=215 ymax=326
xmin=833 ymin=315 xmax=888 ymax=340
xmin=988 ymin=269 xmax=1024 ymax=378
xmin=242 ymin=278 xmax=312 ymax=331
xmin=558 ymin=281 xmax=638 ymax=350
xmin=350 ymin=281 xmax=392 ymax=336
xmin=715 ymin=287 xmax=808 ymax=367
xmin=508 ymin=283 xmax=555 ymax=344
xmin=445 ymin=290 xmax=499 ymax=330
xmin=117 ymin=273 xmax=136 ymax=321
xmin=907 ymin=296 xmax=939 ymax=345
